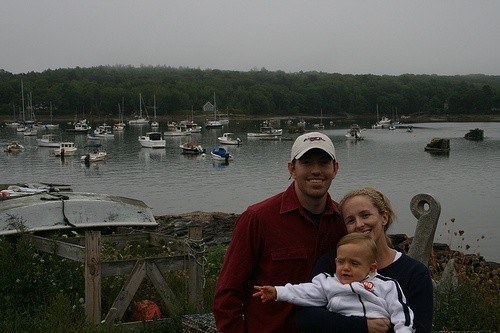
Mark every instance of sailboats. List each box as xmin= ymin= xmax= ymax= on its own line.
xmin=2 ymin=77 xmax=60 ymax=136
xmin=112 ymin=96 xmax=127 ymax=131
xmin=128 ymin=92 xmax=151 ymax=124
xmin=147 ymin=89 xmax=229 ymax=136
xmin=285 ymin=108 xmax=325 ymax=127
xmin=330 ymin=112 xmax=333 ymax=123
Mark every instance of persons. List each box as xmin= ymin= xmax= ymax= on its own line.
xmin=292 ymin=186 xmax=434 ymax=333
xmin=252 ymin=233 xmax=415 ymax=333
xmin=213 ymin=132 xmax=346 ymax=333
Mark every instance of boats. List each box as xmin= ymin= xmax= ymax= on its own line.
xmin=371 ymin=103 xmax=395 ymax=130
xmin=407 ymin=130 xmax=413 ymax=132
xmin=87 ymin=123 xmax=114 ymax=139
xmin=344 ymin=124 xmax=371 ymax=140
xmin=210 ymin=147 xmax=234 ymax=161
xmin=80 ymin=143 xmax=107 ymax=162
xmin=36 ymin=135 xmax=61 ymax=147
xmin=424 ymin=137 xmax=451 ymax=153
xmin=246 ymin=119 xmax=282 ymax=137
xmin=0 ymin=191 xmax=160 ymax=235
xmin=138 ymin=132 xmax=166 ymax=147
xmin=54 ymin=142 xmax=78 ymax=157
xmin=259 ymin=137 xmax=293 ymax=141
xmin=179 ymin=144 xmax=207 ymax=154
xmin=6 ymin=141 xmax=25 ymax=150
xmin=217 ymin=133 xmax=244 ymax=144
xmin=65 ymin=118 xmax=92 ymax=133
xmin=464 ymin=128 xmax=484 ymax=140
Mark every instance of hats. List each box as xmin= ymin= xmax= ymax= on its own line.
xmin=290 ymin=132 xmax=336 ymax=163
